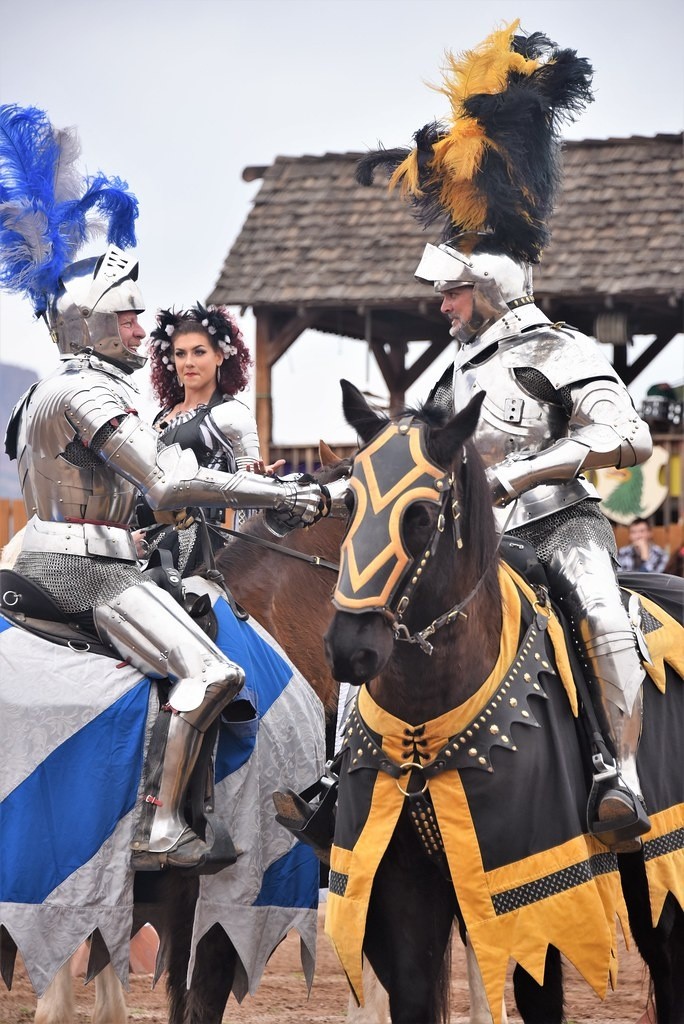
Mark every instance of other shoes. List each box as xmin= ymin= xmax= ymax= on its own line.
xmin=599 ymin=790 xmax=642 ymax=854
xmin=273 ymin=787 xmax=337 ymax=865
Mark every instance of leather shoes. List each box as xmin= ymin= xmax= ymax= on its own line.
xmin=130 ymin=837 xmax=244 ymax=871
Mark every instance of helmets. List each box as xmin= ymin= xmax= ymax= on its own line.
xmin=42 ymin=243 xmax=148 ymax=395
xmin=414 ymin=229 xmax=554 ymax=372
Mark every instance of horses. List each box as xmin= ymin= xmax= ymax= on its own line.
xmin=1 ymin=376 xmax=684 ymax=1024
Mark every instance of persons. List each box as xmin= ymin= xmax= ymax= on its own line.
xmin=127 ymin=320 xmax=287 ymax=576
xmin=272 ymin=227 xmax=652 ymax=855
xmin=0 ymin=253 xmax=246 ymax=872
xmin=617 ymin=518 xmax=669 ymax=573
